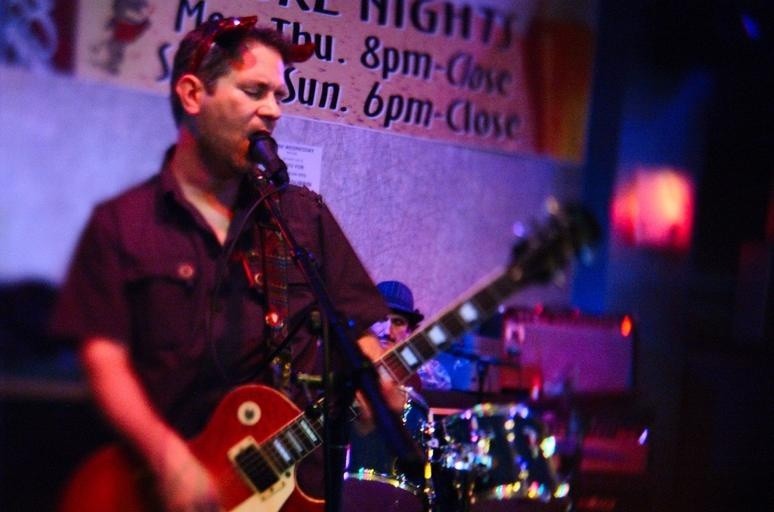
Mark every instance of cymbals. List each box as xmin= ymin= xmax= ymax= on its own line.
xmin=438 ymin=342 xmax=523 ymax=370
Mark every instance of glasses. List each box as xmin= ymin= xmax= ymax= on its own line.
xmin=188 ymin=16 xmax=258 ymax=72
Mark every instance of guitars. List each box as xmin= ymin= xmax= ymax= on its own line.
xmin=58 ymin=196 xmax=604 ymax=512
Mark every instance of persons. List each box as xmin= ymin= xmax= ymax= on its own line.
xmin=346 ymin=279 xmax=428 ymax=479
xmin=47 ymin=16 xmax=391 ymax=512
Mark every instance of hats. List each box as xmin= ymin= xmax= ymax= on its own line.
xmin=377 ymin=281 xmax=415 ymax=314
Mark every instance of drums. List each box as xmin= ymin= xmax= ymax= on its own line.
xmin=426 ymin=401 xmax=573 ymax=511
xmin=342 ymin=382 xmax=436 ymax=512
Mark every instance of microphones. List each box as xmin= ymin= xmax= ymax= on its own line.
xmin=243 ymin=130 xmax=292 ymax=184
